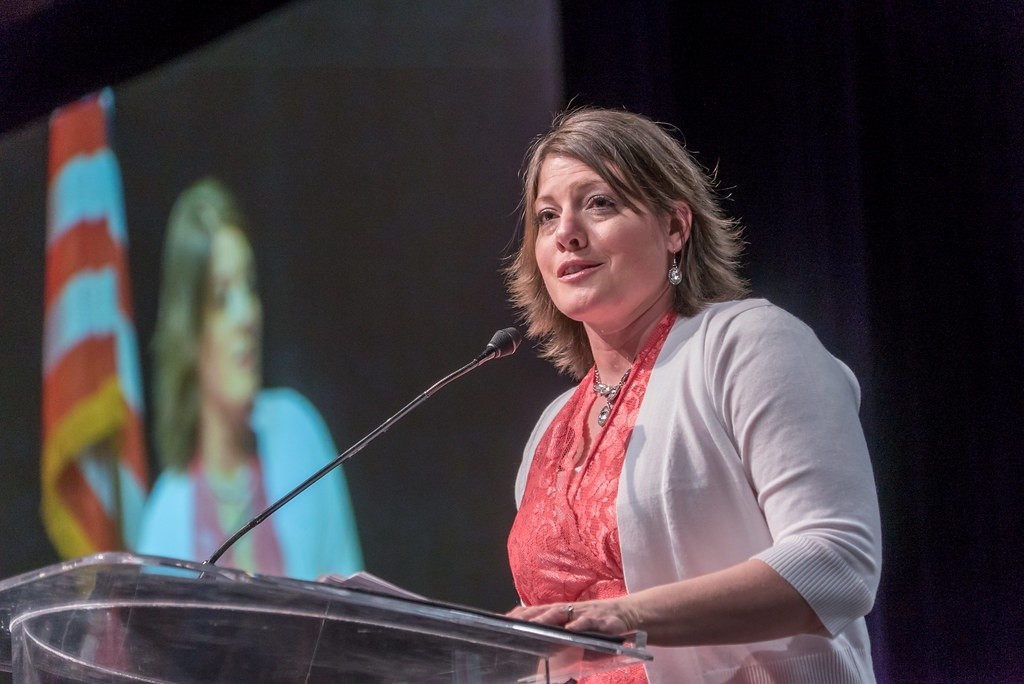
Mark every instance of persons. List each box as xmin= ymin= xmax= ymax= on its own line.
xmin=138 ymin=179 xmax=368 ymax=583
xmin=503 ymin=110 xmax=883 ymax=684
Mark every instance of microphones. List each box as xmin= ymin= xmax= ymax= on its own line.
xmin=210 ymin=327 xmax=523 ymax=567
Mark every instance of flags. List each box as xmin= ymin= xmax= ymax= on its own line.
xmin=39 ymin=86 xmax=148 ymax=599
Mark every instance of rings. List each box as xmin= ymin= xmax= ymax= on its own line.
xmin=568 ymin=604 xmax=574 ymax=623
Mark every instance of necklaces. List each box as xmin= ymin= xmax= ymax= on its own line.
xmin=201 ymin=461 xmax=253 ymax=501
xmin=592 ymin=354 xmax=639 ymax=427
